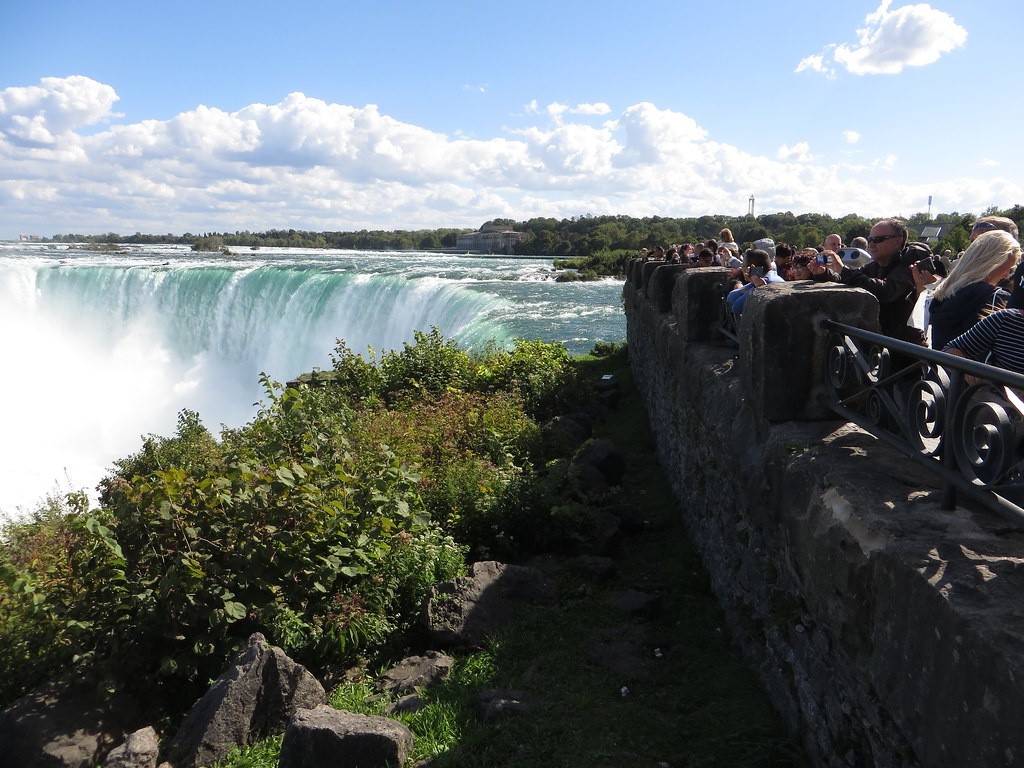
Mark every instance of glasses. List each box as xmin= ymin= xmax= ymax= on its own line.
xmin=867 ymin=234 xmax=896 ymax=243
xmin=702 ymin=256 xmax=714 ymax=266
xmin=738 ymin=264 xmax=748 ymax=269
xmin=971 ymin=221 xmax=997 ymax=232
xmin=790 ymin=267 xmax=809 ymax=274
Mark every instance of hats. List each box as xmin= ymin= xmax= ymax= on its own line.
xmin=753 ymin=238 xmax=776 ymax=262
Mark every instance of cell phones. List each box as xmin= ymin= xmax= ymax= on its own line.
xmin=815 ymin=253 xmax=827 ymax=264
xmin=917 ymin=257 xmax=936 ymax=275
xmin=749 ymin=267 xmax=764 ymax=277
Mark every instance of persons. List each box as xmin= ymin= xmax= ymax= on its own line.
xmin=638 ymin=216 xmax=1023 ymax=401
